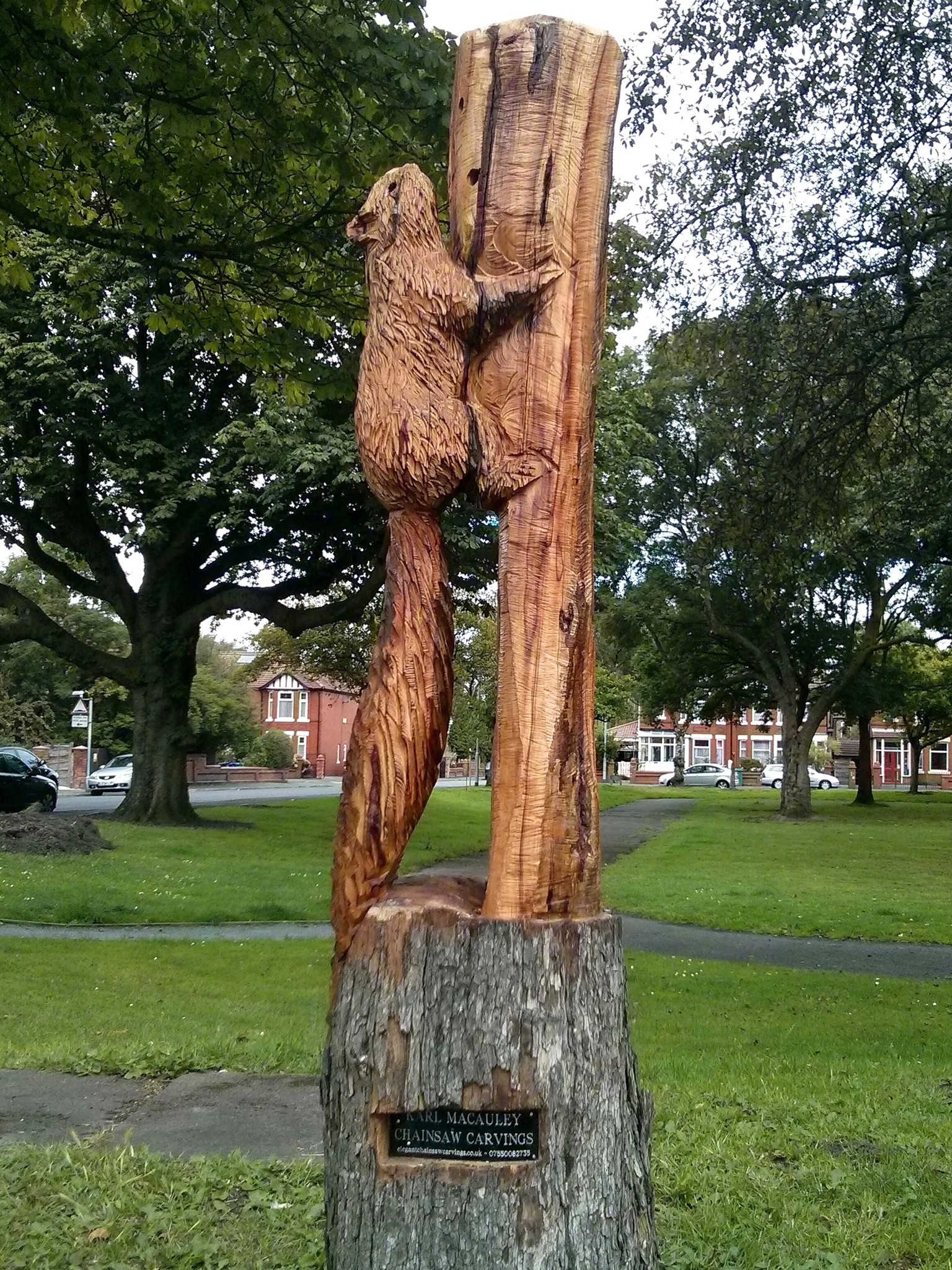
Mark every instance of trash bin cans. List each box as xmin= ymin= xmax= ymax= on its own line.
xmin=735 ymin=768 xmax=744 ymax=786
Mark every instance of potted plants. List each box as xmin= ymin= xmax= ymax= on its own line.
xmin=739 ymin=757 xmax=763 ymax=785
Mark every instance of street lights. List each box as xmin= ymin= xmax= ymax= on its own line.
xmin=72 ymin=690 xmax=93 ymax=792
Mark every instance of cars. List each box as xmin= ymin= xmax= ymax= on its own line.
xmin=485 ymin=761 xmax=490 ymax=780
xmin=88 ymin=754 xmax=133 ymax=795
xmin=0 ymin=747 xmax=59 ymax=789
xmin=0 ymin=751 xmax=58 ymax=813
xmin=658 ymin=763 xmax=739 ymax=790
xmin=759 ymin=764 xmax=840 ymax=790
